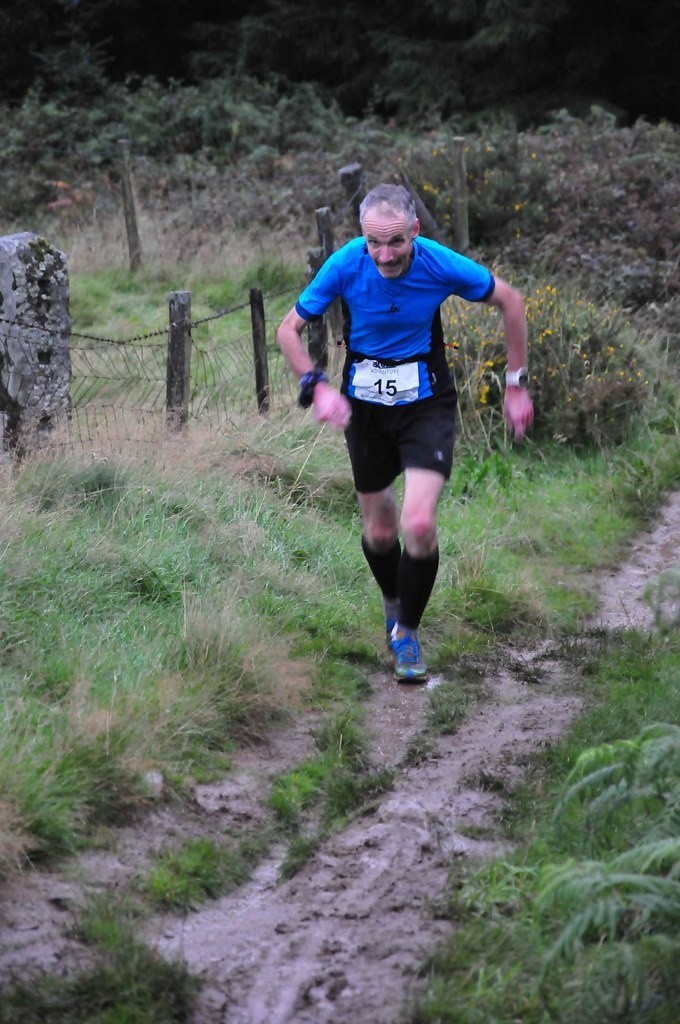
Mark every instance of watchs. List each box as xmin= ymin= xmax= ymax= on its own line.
xmin=506 ymin=366 xmax=530 ymax=388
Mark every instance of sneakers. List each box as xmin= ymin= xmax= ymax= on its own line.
xmin=384 ymin=597 xmax=399 ymax=650
xmin=391 ymin=622 xmax=428 ymax=680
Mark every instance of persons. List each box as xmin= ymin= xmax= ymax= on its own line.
xmin=276 ymin=183 xmax=534 ymax=683
